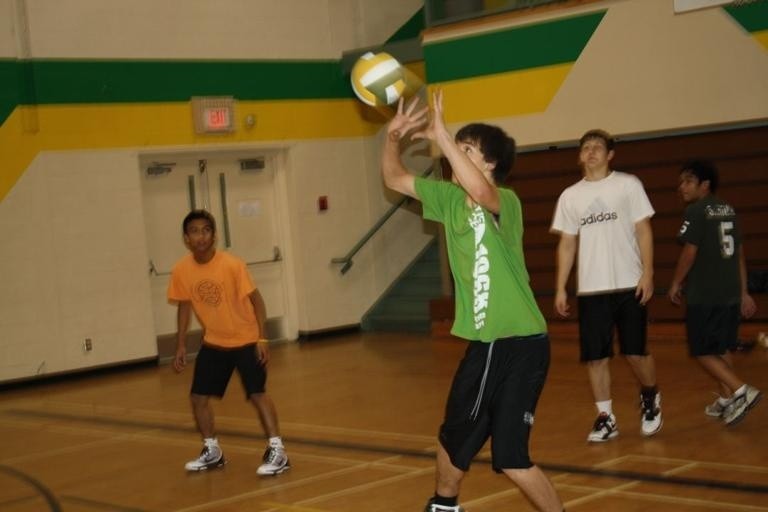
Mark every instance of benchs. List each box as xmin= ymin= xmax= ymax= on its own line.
xmin=612 ymin=125 xmax=768 ymax=346
xmin=428 ymin=297 xmax=469 ymax=346
xmin=504 ymin=146 xmax=585 ymax=346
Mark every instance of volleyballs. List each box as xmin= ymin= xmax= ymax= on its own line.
xmin=350 ymin=49 xmax=409 ymax=108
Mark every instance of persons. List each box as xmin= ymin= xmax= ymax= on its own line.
xmin=668 ymin=158 xmax=764 ymax=428
xmin=550 ymin=126 xmax=665 ymax=444
xmin=381 ymin=87 xmax=562 ymax=512
xmin=167 ymin=209 xmax=292 ymax=477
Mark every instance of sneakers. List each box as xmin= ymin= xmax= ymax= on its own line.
xmin=256 ymin=447 xmax=292 ymax=475
xmin=705 ymin=401 xmax=727 ymax=416
xmin=185 ymin=445 xmax=224 ymax=472
xmin=589 ymin=412 xmax=619 ymax=443
xmin=723 ymin=386 xmax=761 ymax=427
xmin=640 ymin=389 xmax=662 ymax=436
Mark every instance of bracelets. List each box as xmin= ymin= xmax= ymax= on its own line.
xmin=256 ymin=339 xmax=270 ymax=343
